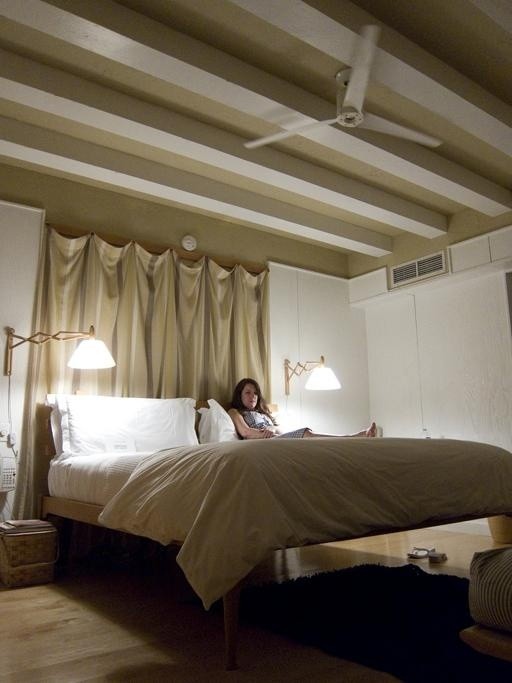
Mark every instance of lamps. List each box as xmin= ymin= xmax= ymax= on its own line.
xmin=3 ymin=326 xmax=117 ymax=377
xmin=284 ymin=356 xmax=343 ymax=395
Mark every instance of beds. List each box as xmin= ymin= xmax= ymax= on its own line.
xmin=36 ymin=399 xmax=512 ymax=671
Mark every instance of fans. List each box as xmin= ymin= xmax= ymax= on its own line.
xmin=243 ymin=23 xmax=444 ymax=155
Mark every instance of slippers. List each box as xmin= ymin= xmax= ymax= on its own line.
xmin=409 ymin=545 xmax=448 ymax=564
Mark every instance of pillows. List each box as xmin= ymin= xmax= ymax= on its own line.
xmin=56 ymin=397 xmax=200 ymax=455
xmin=207 ymin=399 xmax=243 ymax=444
xmin=196 ymin=404 xmax=212 ymax=445
xmin=45 ymin=393 xmax=75 ymax=457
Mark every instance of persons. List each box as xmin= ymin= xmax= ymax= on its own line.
xmin=227 ymin=378 xmax=377 ymax=439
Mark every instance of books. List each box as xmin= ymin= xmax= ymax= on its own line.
xmin=0 ymin=519 xmax=55 ymax=534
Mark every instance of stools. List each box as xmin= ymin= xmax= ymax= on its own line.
xmin=457 ymin=544 xmax=512 ymax=664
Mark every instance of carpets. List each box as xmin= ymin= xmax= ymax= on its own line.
xmin=235 ymin=564 xmax=511 ymax=682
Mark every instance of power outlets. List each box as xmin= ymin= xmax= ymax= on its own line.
xmin=0 ymin=421 xmax=13 ymax=443
